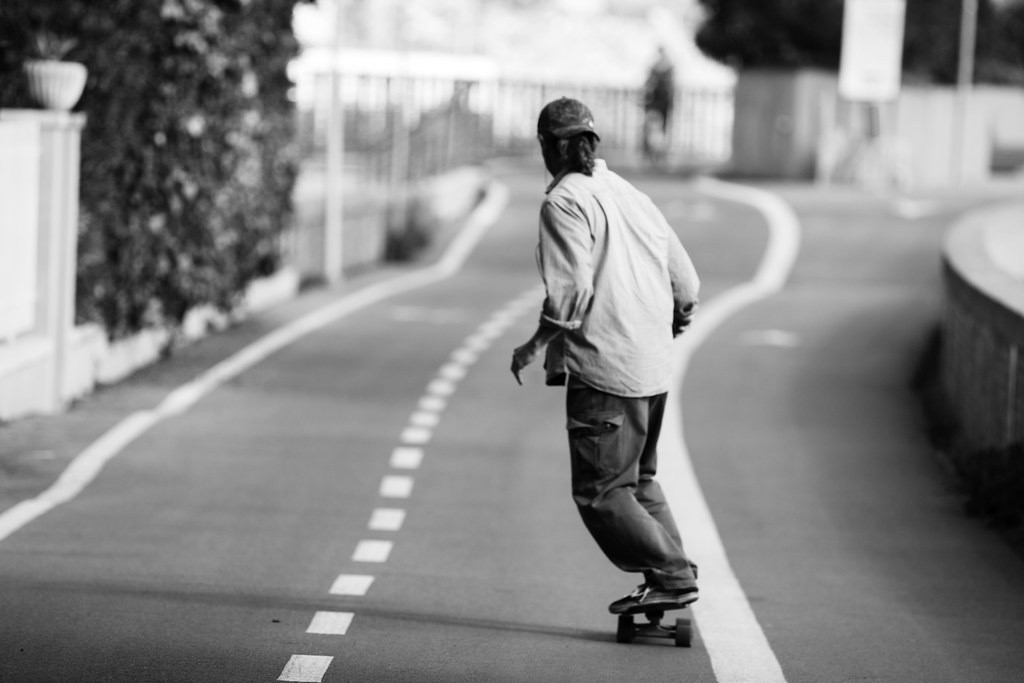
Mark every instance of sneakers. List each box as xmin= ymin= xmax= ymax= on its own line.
xmin=609 ymin=587 xmax=700 ymax=613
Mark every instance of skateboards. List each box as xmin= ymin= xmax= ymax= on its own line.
xmin=616 ymin=600 xmax=694 ymax=648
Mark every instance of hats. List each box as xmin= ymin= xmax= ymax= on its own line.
xmin=537 ymin=98 xmax=601 ymax=141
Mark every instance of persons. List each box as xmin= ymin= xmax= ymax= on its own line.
xmin=508 ymin=93 xmax=704 ymax=618
